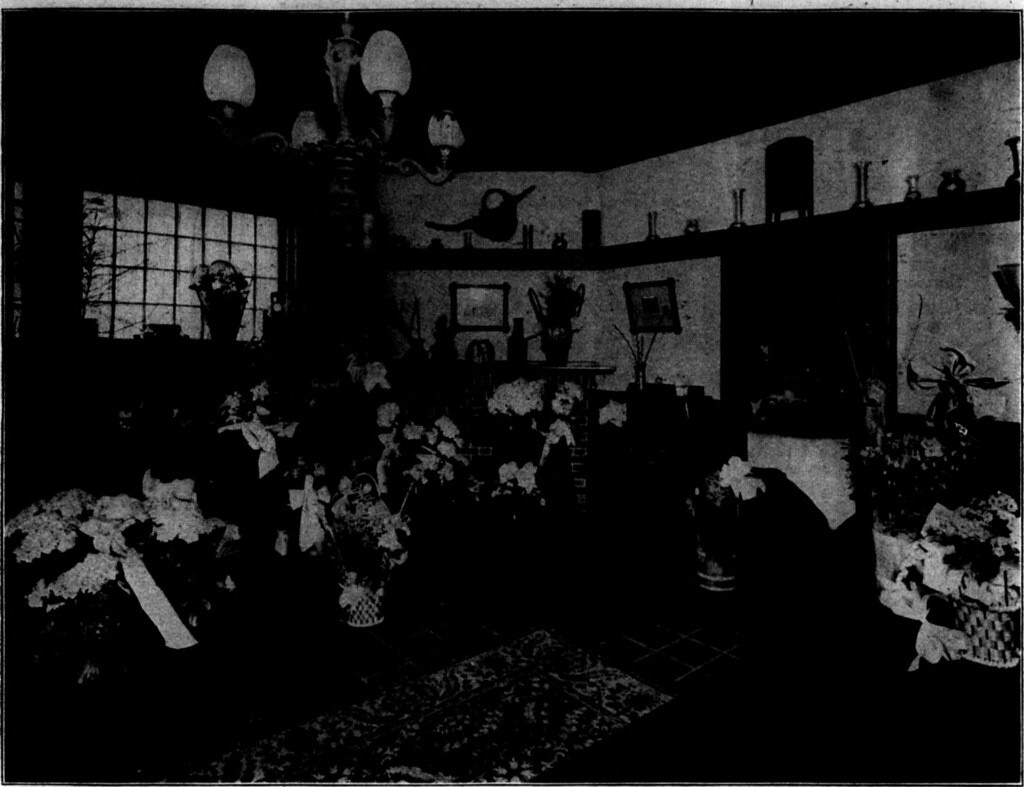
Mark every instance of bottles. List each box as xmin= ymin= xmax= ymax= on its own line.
xmin=853 ymin=161 xmax=871 ymax=210
xmin=646 ymin=212 xmax=660 ymax=240
xmin=463 ymin=232 xmax=474 ymax=248
xmin=903 ymin=175 xmax=920 ymax=202
xmin=729 ymin=188 xmax=746 ymax=227
xmin=935 ymin=172 xmax=965 ymax=194
xmin=552 ymin=232 xmax=568 ymax=249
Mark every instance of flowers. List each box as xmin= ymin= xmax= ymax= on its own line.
xmin=191 ymin=262 xmax=257 ymax=307
xmin=3 ymin=352 xmax=1023 ymax=615
xmin=538 ymin=270 xmax=587 ymax=322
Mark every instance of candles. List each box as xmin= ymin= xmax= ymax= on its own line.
xmin=1 ymin=169 xmax=160 ymax=350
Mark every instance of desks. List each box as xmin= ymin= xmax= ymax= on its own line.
xmin=396 ymin=359 xmax=616 ymax=514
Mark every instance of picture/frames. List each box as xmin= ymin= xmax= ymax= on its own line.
xmin=622 ymin=277 xmax=683 ymax=337
xmin=448 ymin=280 xmax=512 ymax=334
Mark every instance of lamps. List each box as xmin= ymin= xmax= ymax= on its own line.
xmin=202 ymin=9 xmax=464 ymax=210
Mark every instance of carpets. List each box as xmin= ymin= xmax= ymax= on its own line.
xmin=161 ymin=628 xmax=675 ymax=785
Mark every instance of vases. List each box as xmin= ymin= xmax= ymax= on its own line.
xmin=400 ymin=338 xmax=429 ymax=359
xmin=632 ymin=364 xmax=647 ymax=391
xmin=507 ymin=316 xmax=528 ymax=361
